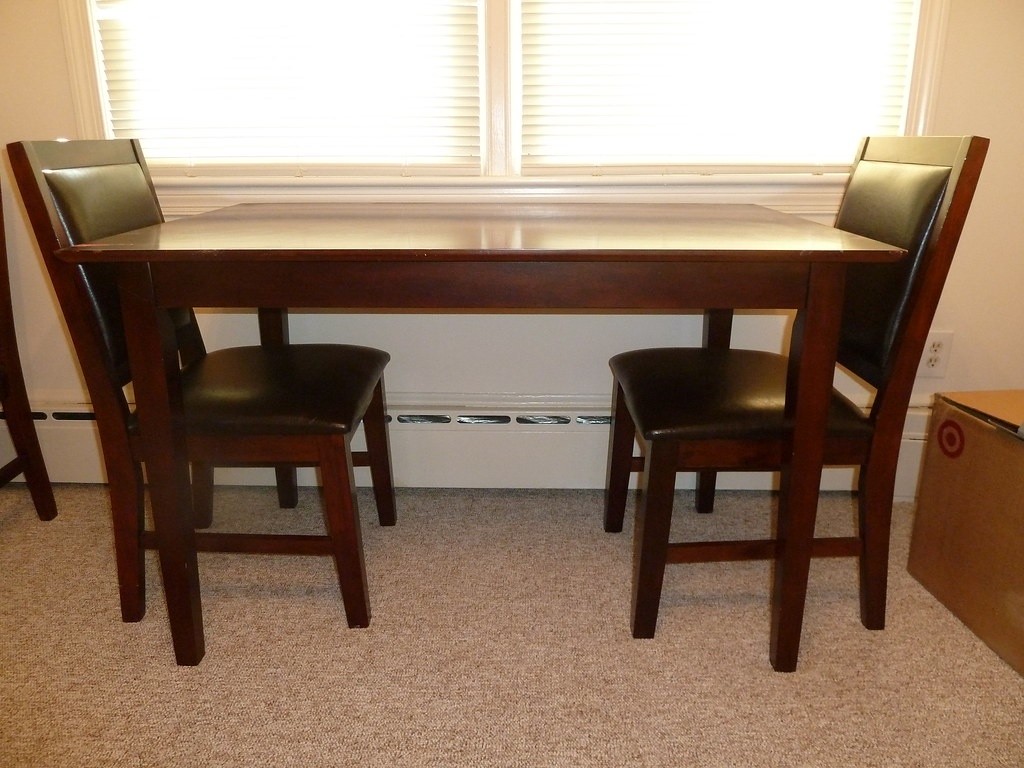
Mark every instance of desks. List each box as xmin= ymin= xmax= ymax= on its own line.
xmin=56 ymin=202 xmax=908 ymax=672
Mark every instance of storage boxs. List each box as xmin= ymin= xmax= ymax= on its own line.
xmin=907 ymin=389 xmax=1023 ymax=678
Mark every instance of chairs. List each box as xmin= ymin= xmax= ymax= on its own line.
xmin=601 ymin=135 xmax=991 ymax=641
xmin=7 ymin=140 xmax=398 ymax=628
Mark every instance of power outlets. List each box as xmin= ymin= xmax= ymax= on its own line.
xmin=918 ymin=331 xmax=952 ymax=377
